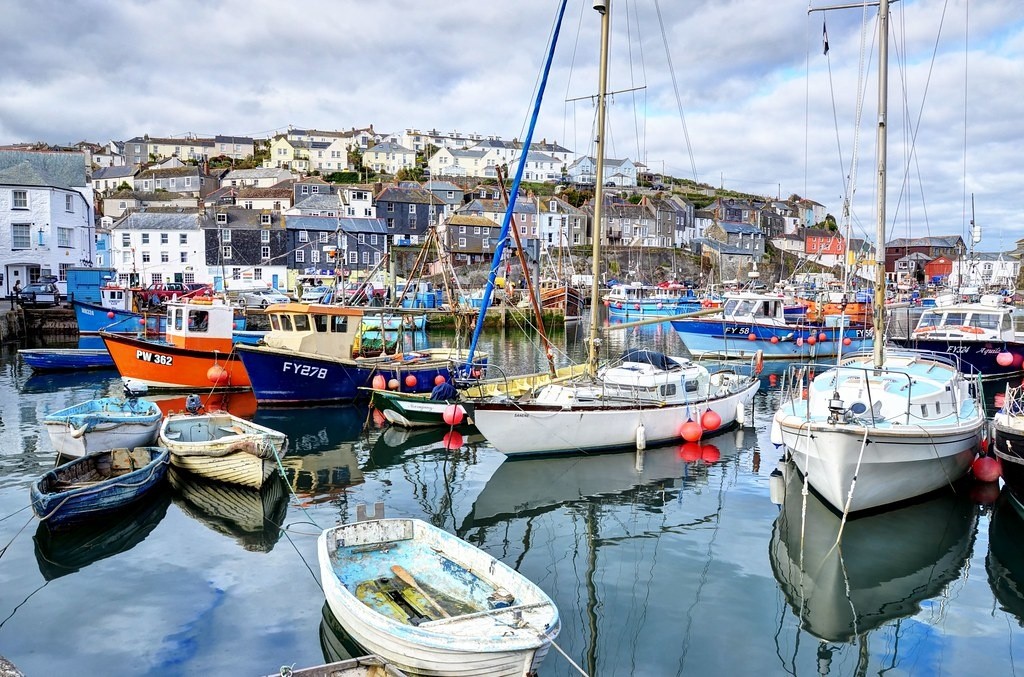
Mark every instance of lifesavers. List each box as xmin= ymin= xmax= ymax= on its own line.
xmin=914 ymin=326 xmax=935 ymax=333
xmin=506 ymin=286 xmax=515 ymax=295
xmin=193 ymin=295 xmax=217 ymax=301
xmin=962 ymin=325 xmax=985 ymax=334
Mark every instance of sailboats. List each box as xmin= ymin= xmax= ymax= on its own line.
xmin=446 ymin=1 xmax=763 ymax=459
xmin=768 ymin=465 xmax=980 ymax=677
xmin=373 ymin=163 xmax=610 ymax=430
xmin=457 ymin=419 xmax=758 ymax=676
xmin=770 ymin=0 xmax=990 ymax=518
xmin=539 ymin=212 xmax=584 ymax=321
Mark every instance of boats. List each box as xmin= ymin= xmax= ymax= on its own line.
xmin=18 ymin=348 xmax=116 ymax=372
xmin=32 ymin=473 xmax=171 ymax=582
xmin=31 ymin=447 xmax=171 ymax=537
xmin=317 ymin=517 xmax=562 ymax=677
xmin=669 ymin=261 xmax=874 ymax=358
xmin=984 ymin=492 xmax=1024 ymax=629
xmin=166 ymin=464 xmax=290 ymax=553
xmin=336 ymin=313 xmax=426 ymax=331
xmin=234 ymin=302 xmax=488 ymax=406
xmin=72 ymin=286 xmax=247 ymax=336
xmin=601 ymin=252 xmax=1017 ymax=326
xmin=370 ymin=425 xmax=487 ymax=470
xmin=250 ymin=399 xmax=374 ymax=496
xmin=45 ymin=398 xmax=163 ymax=461
xmin=883 ymin=193 xmax=1024 ymax=420
xmin=97 ymin=292 xmax=252 ymax=395
xmin=157 ymin=410 xmax=289 ymax=490
xmin=19 ymin=369 xmax=121 ymax=395
xmin=992 ymin=382 xmax=1024 ymax=519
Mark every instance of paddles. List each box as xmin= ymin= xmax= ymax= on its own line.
xmin=390 ymin=565 xmax=451 ymax=619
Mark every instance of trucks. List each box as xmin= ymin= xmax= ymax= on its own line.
xmin=337 ymin=282 xmax=387 ymax=302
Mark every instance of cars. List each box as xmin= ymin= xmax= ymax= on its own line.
xmin=186 ymin=284 xmax=213 ymax=297
xmin=395 ymin=282 xmax=417 ymax=301
xmin=137 ymin=283 xmax=196 ymax=308
xmin=746 ymin=279 xmax=767 ymax=289
xmin=238 ymin=286 xmax=290 ymax=310
xmin=679 ymin=281 xmax=698 ymax=289
xmin=494 ymin=277 xmax=516 ymax=289
xmin=18 ymin=275 xmax=60 ymax=308
xmin=300 ymin=286 xmax=335 ymax=304
